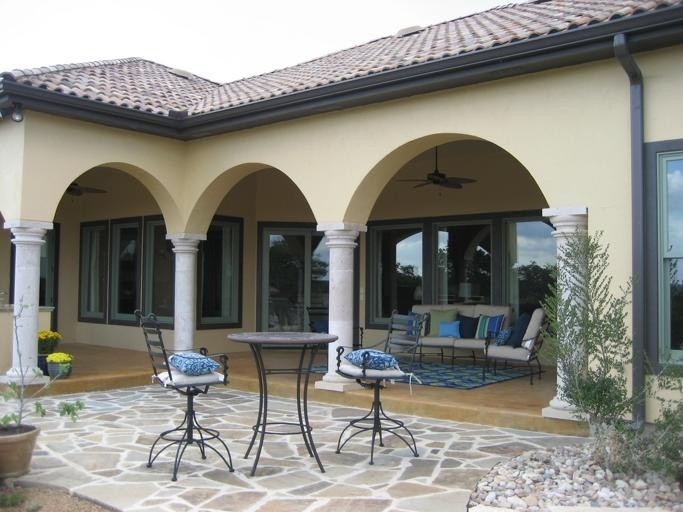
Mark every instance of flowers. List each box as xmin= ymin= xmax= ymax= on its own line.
xmin=38 ymin=330 xmax=64 ymax=354
xmin=45 ymin=352 xmax=74 ymax=364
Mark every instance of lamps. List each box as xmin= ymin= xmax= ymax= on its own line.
xmin=0 ymin=102 xmax=24 ymax=122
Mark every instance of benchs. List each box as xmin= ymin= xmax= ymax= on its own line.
xmin=387 ymin=303 xmax=513 ymax=373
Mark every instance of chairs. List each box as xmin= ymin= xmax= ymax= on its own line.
xmin=483 ymin=307 xmax=553 ymax=386
xmin=335 ymin=308 xmax=430 ymax=466
xmin=134 ymin=308 xmax=234 ymax=482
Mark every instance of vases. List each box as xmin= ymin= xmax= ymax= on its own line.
xmin=47 ymin=361 xmax=72 ymax=380
xmin=37 ymin=354 xmax=48 ymax=376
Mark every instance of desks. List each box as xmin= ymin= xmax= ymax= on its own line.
xmin=225 ymin=332 xmax=339 ymax=477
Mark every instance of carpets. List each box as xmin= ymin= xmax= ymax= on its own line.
xmin=301 ymin=362 xmax=548 ymax=390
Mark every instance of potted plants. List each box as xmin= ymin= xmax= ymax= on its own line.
xmin=0 ymin=284 xmax=86 ymax=479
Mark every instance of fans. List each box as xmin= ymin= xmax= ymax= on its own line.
xmin=65 ymin=182 xmax=111 ymax=196
xmin=394 ymin=146 xmax=477 ymax=190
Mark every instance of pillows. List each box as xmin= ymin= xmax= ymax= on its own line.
xmin=343 ymin=348 xmax=400 ymax=370
xmin=406 ymin=309 xmax=531 ymax=348
xmin=167 ymin=352 xmax=222 ymax=376
